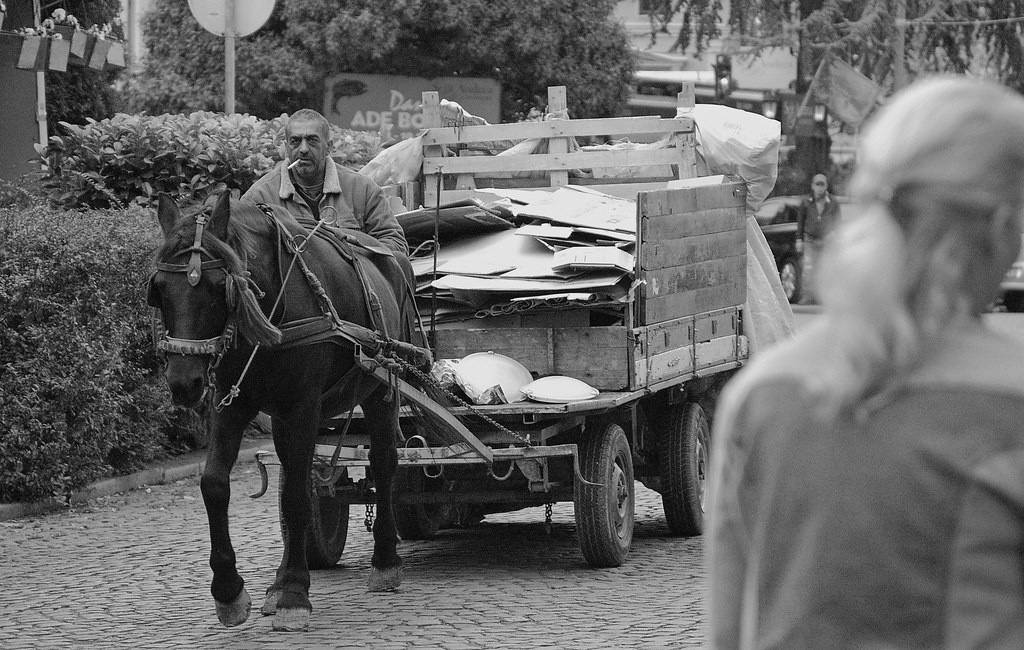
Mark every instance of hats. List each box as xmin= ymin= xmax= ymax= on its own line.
xmin=813 ymin=173 xmax=828 ymax=187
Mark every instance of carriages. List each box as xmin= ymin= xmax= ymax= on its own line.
xmin=146 ymin=79 xmax=752 ymax=633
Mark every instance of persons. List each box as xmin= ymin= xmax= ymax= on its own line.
xmin=239 ymin=108 xmax=417 ymax=407
xmin=795 ymin=174 xmax=842 ymax=305
xmin=702 ymin=76 xmax=1023 ymax=650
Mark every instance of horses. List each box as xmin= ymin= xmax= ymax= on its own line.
xmin=145 ymin=186 xmax=478 ymax=631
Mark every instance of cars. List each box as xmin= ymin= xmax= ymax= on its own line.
xmin=754 ymin=196 xmax=849 ymax=305
